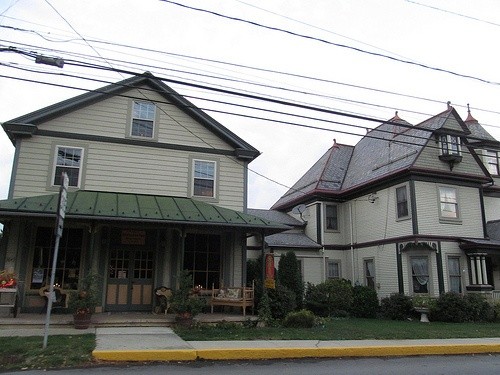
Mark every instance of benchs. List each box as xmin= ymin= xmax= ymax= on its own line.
xmin=22 ymin=294 xmax=67 ymax=314
xmin=211 ymin=279 xmax=254 ymax=317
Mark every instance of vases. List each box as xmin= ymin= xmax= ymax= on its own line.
xmin=0 ymin=288 xmax=17 ymax=308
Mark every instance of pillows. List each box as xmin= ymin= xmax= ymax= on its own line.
xmin=217 ymin=288 xmax=240 ymax=298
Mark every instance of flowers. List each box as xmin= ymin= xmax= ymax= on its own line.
xmin=0 ymin=270 xmax=17 ymax=288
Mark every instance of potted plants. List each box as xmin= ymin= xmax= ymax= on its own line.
xmin=172 ymin=270 xmax=205 ymax=328
xmin=68 ymin=272 xmax=103 ymax=329
xmin=411 ymin=293 xmax=437 ymax=322
